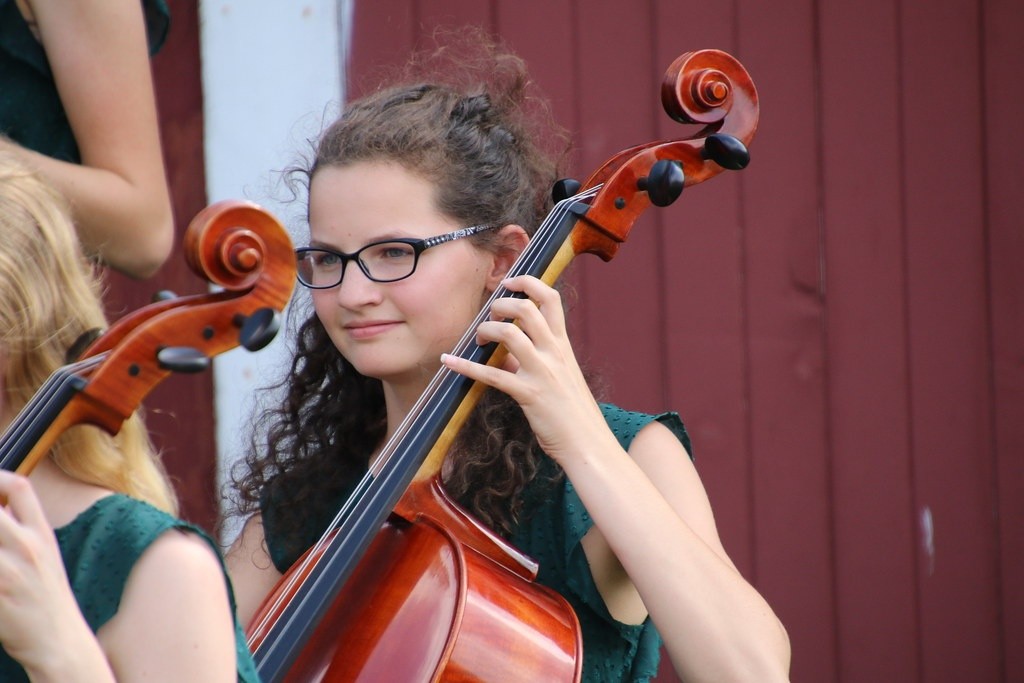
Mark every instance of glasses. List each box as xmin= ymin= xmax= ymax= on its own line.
xmin=295 ymin=223 xmax=496 ymax=289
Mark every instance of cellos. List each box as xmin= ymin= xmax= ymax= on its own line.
xmin=239 ymin=47 xmax=761 ymax=683
xmin=2 ymin=197 xmax=302 ymax=494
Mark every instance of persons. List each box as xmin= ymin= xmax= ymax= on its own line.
xmin=213 ymin=54 xmax=792 ymax=683
xmin=0 ymin=158 xmax=260 ymax=683
xmin=0 ymin=0 xmax=174 ymax=279
